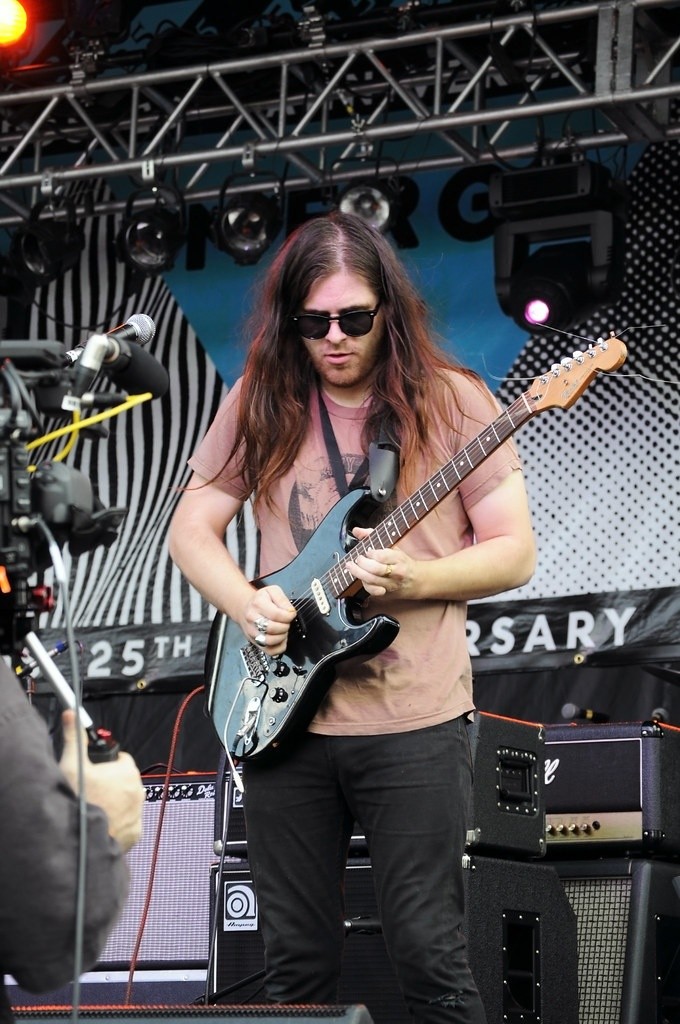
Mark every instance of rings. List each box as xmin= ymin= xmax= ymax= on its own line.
xmin=384 ymin=563 xmax=392 ymax=577
xmin=255 ymin=633 xmax=267 ymax=648
xmin=254 ymin=617 xmax=270 ymax=633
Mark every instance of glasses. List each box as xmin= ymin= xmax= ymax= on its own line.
xmin=292 ymin=297 xmax=383 ymax=341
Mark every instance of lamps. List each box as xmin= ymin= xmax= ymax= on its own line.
xmin=112 ymin=167 xmax=188 ymax=271
xmin=488 ymin=159 xmax=629 ymax=335
xmin=208 ymin=161 xmax=291 ymax=268
xmin=9 ymin=195 xmax=89 ymax=285
xmin=327 ymin=141 xmax=421 ymax=239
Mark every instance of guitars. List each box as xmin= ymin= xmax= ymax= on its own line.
xmin=204 ymin=336 xmax=630 ymax=758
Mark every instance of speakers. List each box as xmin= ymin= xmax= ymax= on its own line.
xmin=537 ymin=856 xmax=680 ymax=1024
xmin=209 ymin=856 xmax=580 ymax=1024
xmin=91 ymin=780 xmax=216 ymax=972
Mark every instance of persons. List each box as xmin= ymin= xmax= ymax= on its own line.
xmin=0 ymin=652 xmax=145 ymax=1024
xmin=166 ymin=214 xmax=535 ymax=1024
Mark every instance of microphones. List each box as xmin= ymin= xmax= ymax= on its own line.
xmin=66 ymin=313 xmax=156 ymax=362
xmin=73 ymin=334 xmax=169 ymax=401
